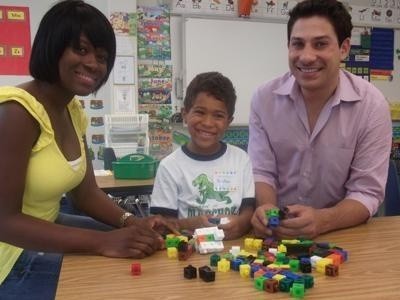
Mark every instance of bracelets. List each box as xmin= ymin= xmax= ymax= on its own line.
xmin=118 ymin=212 xmax=134 ymax=227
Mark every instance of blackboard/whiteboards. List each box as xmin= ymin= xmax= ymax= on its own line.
xmin=180 ymin=13 xmax=400 ymax=127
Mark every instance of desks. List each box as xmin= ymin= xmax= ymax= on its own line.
xmin=56 ymin=215 xmax=400 ymax=299
xmin=94 ymin=167 xmax=158 ymax=218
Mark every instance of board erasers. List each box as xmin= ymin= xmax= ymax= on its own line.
xmin=175 ymin=79 xmax=183 ymax=101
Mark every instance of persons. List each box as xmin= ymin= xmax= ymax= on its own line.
xmin=147 ymin=70 xmax=255 ymax=241
xmin=0 ymin=1 xmax=185 ymax=300
xmin=244 ymin=0 xmax=393 ymax=247
